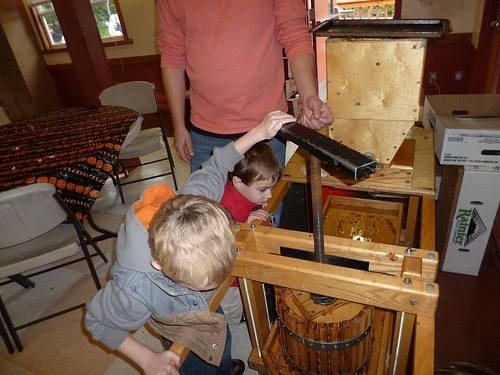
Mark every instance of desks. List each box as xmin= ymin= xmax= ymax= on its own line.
xmin=0 ymin=105 xmax=144 ymax=240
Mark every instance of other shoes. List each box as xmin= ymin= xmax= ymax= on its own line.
xmin=229 ymin=358 xmax=246 ymax=375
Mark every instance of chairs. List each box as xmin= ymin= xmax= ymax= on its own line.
xmin=99 ymin=80 xmax=178 ymax=204
xmin=0 ymin=183 xmax=109 ymax=353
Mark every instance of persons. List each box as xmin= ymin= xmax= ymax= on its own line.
xmin=84 ymin=110 xmax=298 ymax=375
xmin=155 ymin=1 xmax=334 ymax=320
xmin=114 ymin=17 xmax=122 ymax=35
xmin=49 ymin=27 xmax=60 ymax=43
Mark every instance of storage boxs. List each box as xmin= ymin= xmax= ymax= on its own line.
xmin=436 ymin=165 xmax=500 ymax=276
xmin=423 ymin=94 xmax=500 ymax=167
